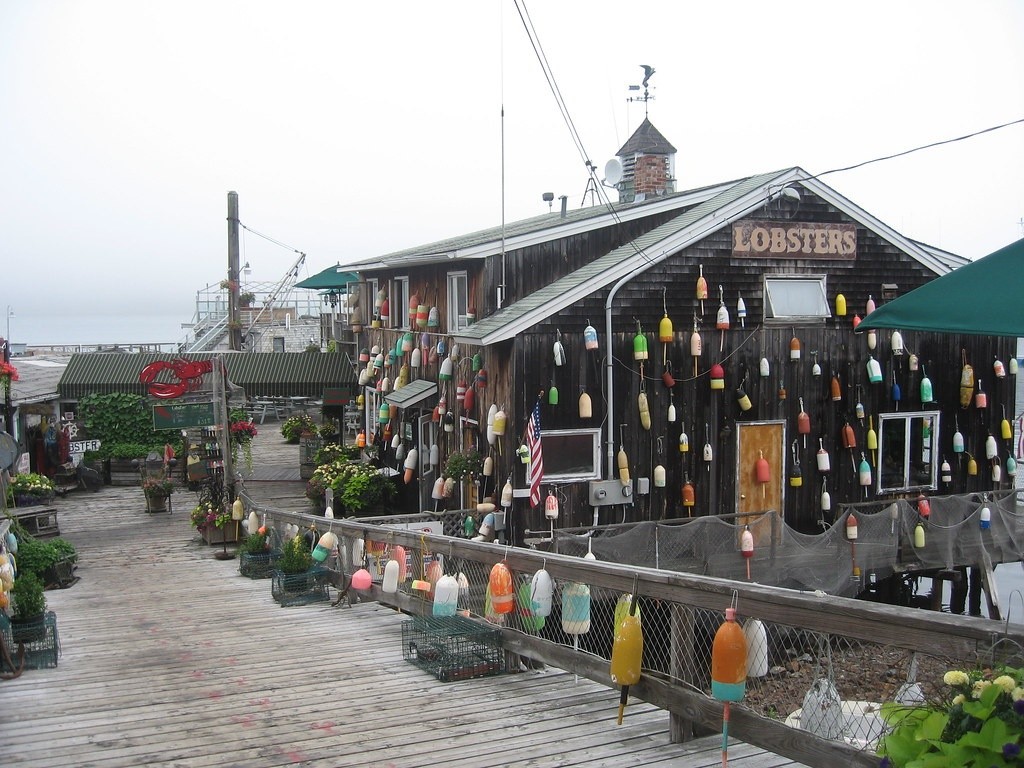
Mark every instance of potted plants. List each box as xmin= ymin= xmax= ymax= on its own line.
xmin=110 ymin=444 xmax=146 ymax=473
xmin=19 ymin=537 xmax=80 ymax=587
xmin=301 ymin=424 xmax=317 ymax=437
xmin=272 ymin=540 xmax=312 ymax=591
xmin=84 ymin=449 xmax=107 ymax=472
xmin=10 ymin=569 xmax=47 ymax=643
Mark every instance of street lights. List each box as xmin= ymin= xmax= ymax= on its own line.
xmin=226 ymin=261 xmax=253 ymax=352
xmin=5 ymin=304 xmax=18 ymax=343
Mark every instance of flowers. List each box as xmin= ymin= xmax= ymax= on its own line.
xmin=280 ymin=414 xmax=311 ymax=442
xmin=0 ymin=360 xmax=19 ymax=390
xmin=190 ymin=500 xmax=231 ymax=532
xmin=238 ymin=525 xmax=270 ymax=566
xmin=6 ymin=473 xmax=58 ymax=496
xmin=305 ymin=441 xmax=397 ymax=510
xmin=231 ymin=420 xmax=257 ymax=476
xmin=143 ymin=477 xmax=182 ymax=496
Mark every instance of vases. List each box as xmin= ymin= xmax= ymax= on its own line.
xmin=12 ymin=490 xmax=54 ymax=506
xmin=145 ymin=493 xmax=167 ymax=509
xmin=310 ymin=495 xmax=385 ymax=518
xmin=201 ymin=521 xmax=235 ymax=546
xmin=290 ymin=427 xmax=302 ymax=442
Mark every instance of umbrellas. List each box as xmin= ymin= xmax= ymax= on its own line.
xmin=318 ymin=287 xmax=353 ymax=313
xmin=855 ymin=238 xmax=1024 ymax=339
xmin=293 ymin=261 xmax=359 ymax=313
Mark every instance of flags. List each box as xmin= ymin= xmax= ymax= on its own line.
xmin=524 ymin=398 xmax=544 ymax=507
